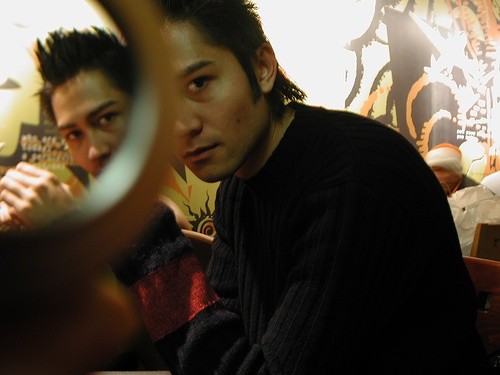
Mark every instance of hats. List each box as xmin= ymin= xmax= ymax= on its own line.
xmin=424 ymin=143 xmax=462 ymax=179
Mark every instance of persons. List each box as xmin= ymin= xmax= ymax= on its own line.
xmin=0 ymin=26 xmax=136 ymax=233
xmin=114 ymin=0 xmax=479 ymax=374
xmin=424 ymin=143 xmax=479 ymax=198
